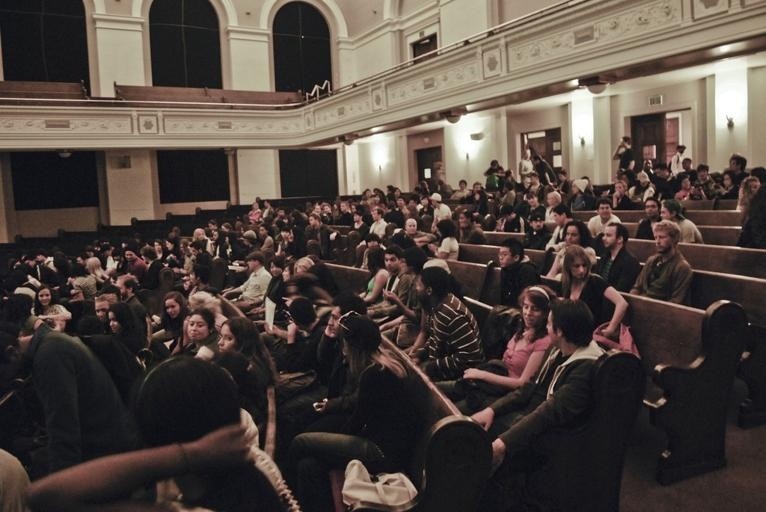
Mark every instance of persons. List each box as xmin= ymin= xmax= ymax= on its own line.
xmin=736 ymin=175 xmax=766 ymax=250
xmin=2 ymin=180 xmax=703 ymax=510
xmin=483 ymin=148 xmax=569 ymax=189
xmin=613 ymin=137 xmax=766 ymax=200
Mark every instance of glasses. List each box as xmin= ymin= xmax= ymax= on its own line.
xmin=338 ymin=310 xmax=360 ymax=331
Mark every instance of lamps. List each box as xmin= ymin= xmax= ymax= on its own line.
xmin=726 ymin=114 xmax=736 ymax=129
xmin=577 ymin=135 xmax=586 ymax=146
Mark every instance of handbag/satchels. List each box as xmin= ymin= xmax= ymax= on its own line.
xmin=343 ymin=459 xmax=417 ymax=506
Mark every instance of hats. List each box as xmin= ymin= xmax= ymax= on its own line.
xmin=428 ymin=193 xmax=442 ymax=202
xmin=247 ymin=250 xmax=265 ymax=261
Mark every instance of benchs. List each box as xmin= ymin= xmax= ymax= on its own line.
xmin=111 ymin=80 xmax=306 ymax=111
xmin=2 ymin=78 xmax=93 ymax=109
xmin=0 ymin=198 xmax=765 ymax=511
